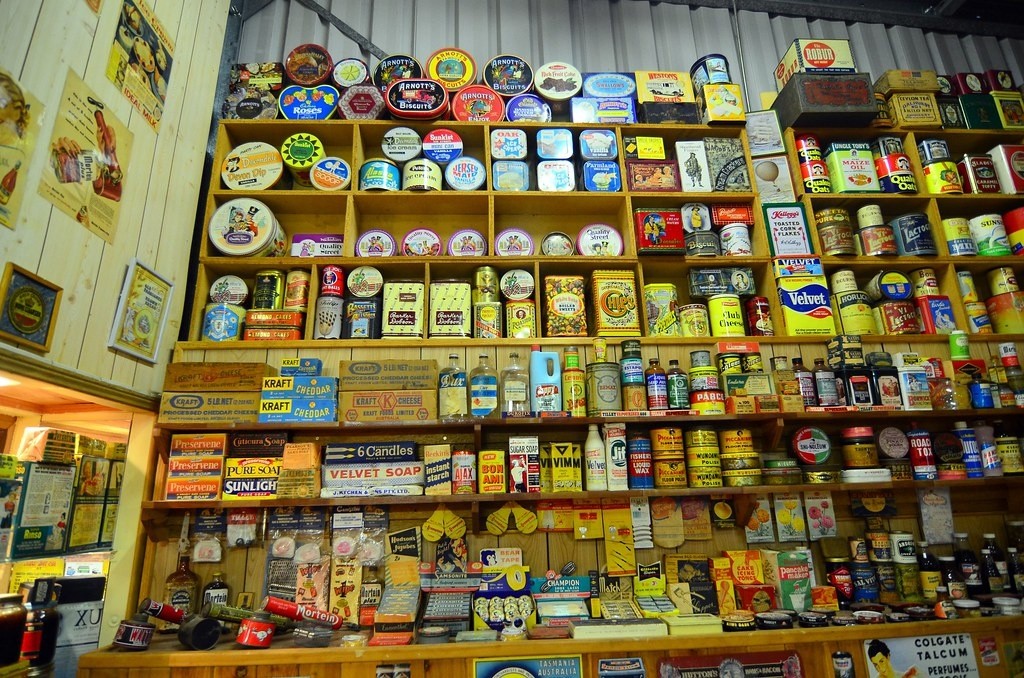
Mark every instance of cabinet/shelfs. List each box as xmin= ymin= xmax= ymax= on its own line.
xmin=76 ymin=57 xmax=1024 ymax=678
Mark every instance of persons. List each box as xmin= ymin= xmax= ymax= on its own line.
xmin=868 ymin=639 xmax=923 ymax=678
xmin=81 ymin=461 xmax=91 ymax=482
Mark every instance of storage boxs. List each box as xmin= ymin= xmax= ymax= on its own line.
xmin=154 ymin=359 xmax=440 ymax=427
xmin=841 ymin=470 xmax=890 ymax=484
xmin=774 ymin=36 xmax=1024 ymax=130
xmin=634 ymin=207 xmax=688 ymax=257
xmin=0 ymin=428 xmax=126 ymax=595
xmin=533 ymin=613 xmax=723 ymax=639
xmin=771 ymin=260 xmax=837 ymax=337
xmin=430 ymin=281 xmax=473 ymax=339
xmin=958 ymin=144 xmax=1024 ymax=194
xmin=322 ymin=443 xmax=426 ymax=497
xmin=163 ymin=434 xmax=284 ymax=500
xmin=292 ymin=233 xmax=344 ymax=257
xmin=625 ymin=135 xmax=750 ymax=192
xmin=592 ymin=269 xmax=641 ymax=335
xmin=546 ymin=277 xmax=587 ymax=336
xmin=382 ymin=281 xmax=424 ymax=337
xmin=686 ymin=267 xmax=754 ymax=295
xmin=823 ymin=140 xmax=881 ymax=193
xmin=571 ymin=71 xmax=747 ymax=125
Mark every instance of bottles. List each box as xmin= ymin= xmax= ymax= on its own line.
xmin=584 ymin=424 xmax=607 ymax=491
xmin=933 ymin=587 xmax=958 ymax=619
xmin=55 ymin=150 xmax=109 ymax=183
xmin=952 ymin=533 xmax=982 ymax=595
xmin=982 ymin=533 xmax=1011 ymax=593
xmin=645 ymin=358 xmax=690 ymax=410
xmin=917 ymin=541 xmax=941 ymax=601
xmin=981 ymin=549 xmax=1003 ymax=595
xmin=939 ymin=556 xmax=967 ymax=600
xmin=792 ymin=358 xmax=839 ymax=407
xmin=0 ymin=593 xmax=27 ymax=667
xmin=159 ymin=543 xmax=197 ymax=634
xmin=358 ymin=566 xmax=384 ymax=629
xmin=438 ymin=345 xmax=562 ymax=419
xmin=0 ymin=160 xmax=21 ymax=205
xmin=968 ymin=354 xmax=1024 ymax=409
xmin=906 ymin=419 xmax=1024 ymax=481
xmin=201 ymin=572 xmax=228 ymax=633
xmin=1007 ymin=548 xmax=1024 ymax=594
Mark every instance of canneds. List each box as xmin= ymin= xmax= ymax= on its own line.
xmin=452 ymin=351 xmax=764 ymax=494
xmin=840 ymin=427 xmax=1024 ymax=481
xmin=825 ymin=529 xmax=923 ymax=605
xmin=798 ymin=132 xmax=1024 ymax=340
xmin=200 ymin=264 xmax=536 ymax=343
xmin=545 ymin=262 xmax=774 ymax=415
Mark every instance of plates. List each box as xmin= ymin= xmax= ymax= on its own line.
xmin=122 ymin=2 xmax=144 ymax=36
xmin=134 ymin=37 xmax=154 ymax=72
xmin=156 ymin=49 xmax=166 ymax=72
xmin=151 ymin=73 xmax=168 ymax=105
xmin=132 ymin=64 xmax=151 ymax=91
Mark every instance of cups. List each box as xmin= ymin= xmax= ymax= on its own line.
xmin=119 ymin=26 xmax=133 ymax=55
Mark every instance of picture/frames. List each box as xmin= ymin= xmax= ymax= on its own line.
xmin=110 ymin=258 xmax=179 ymax=365
xmin=0 ymin=263 xmax=63 ymax=353
